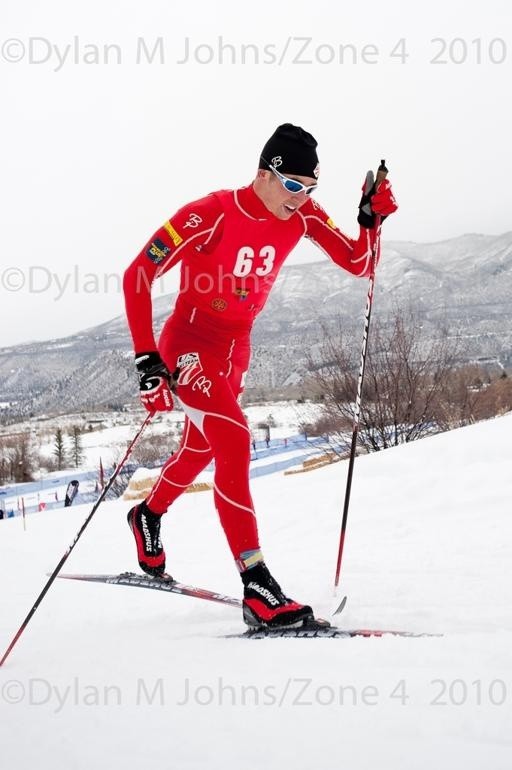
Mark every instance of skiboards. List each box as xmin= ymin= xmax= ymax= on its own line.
xmin=52 ymin=574 xmax=450 ymax=638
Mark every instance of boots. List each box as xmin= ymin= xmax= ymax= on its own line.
xmin=240 ymin=561 xmax=313 ymax=628
xmin=127 ymin=499 xmax=167 ymax=577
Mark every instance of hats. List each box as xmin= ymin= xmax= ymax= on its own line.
xmin=258 ymin=123 xmax=319 ymax=180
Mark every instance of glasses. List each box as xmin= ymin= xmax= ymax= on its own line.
xmin=260 ymin=156 xmax=318 ymax=196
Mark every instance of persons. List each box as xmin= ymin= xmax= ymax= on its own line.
xmin=120 ymin=122 xmax=400 ymax=630
xmin=264 ymin=436 xmax=272 ymax=447
xmin=251 ymin=439 xmax=257 ymax=451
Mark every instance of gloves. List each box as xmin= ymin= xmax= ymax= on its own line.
xmin=357 ymin=170 xmax=397 ymax=229
xmin=135 ymin=351 xmax=179 ymax=418
